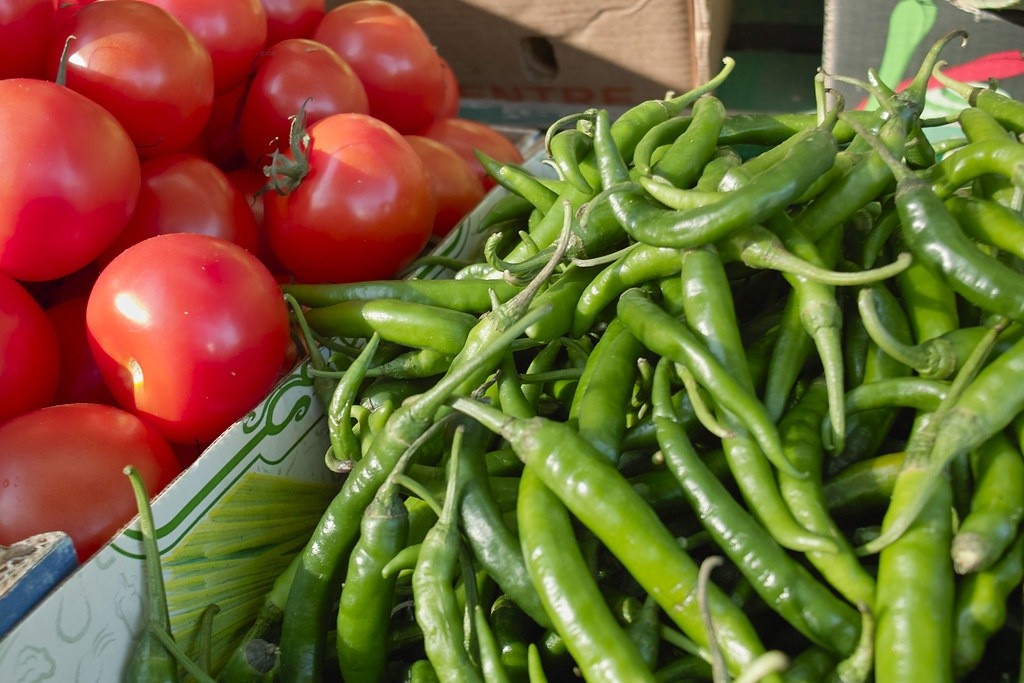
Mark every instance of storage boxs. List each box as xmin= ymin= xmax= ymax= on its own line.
xmin=0 ymin=123 xmax=554 ymax=683
xmin=387 ymin=0 xmax=734 ymax=109
xmin=822 ymin=0 xmax=1024 ymax=111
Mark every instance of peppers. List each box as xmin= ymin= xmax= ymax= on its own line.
xmin=122 ymin=32 xmax=1024 ymax=683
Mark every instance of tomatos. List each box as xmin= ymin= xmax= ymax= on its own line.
xmin=0 ymin=0 xmax=525 ymax=559
